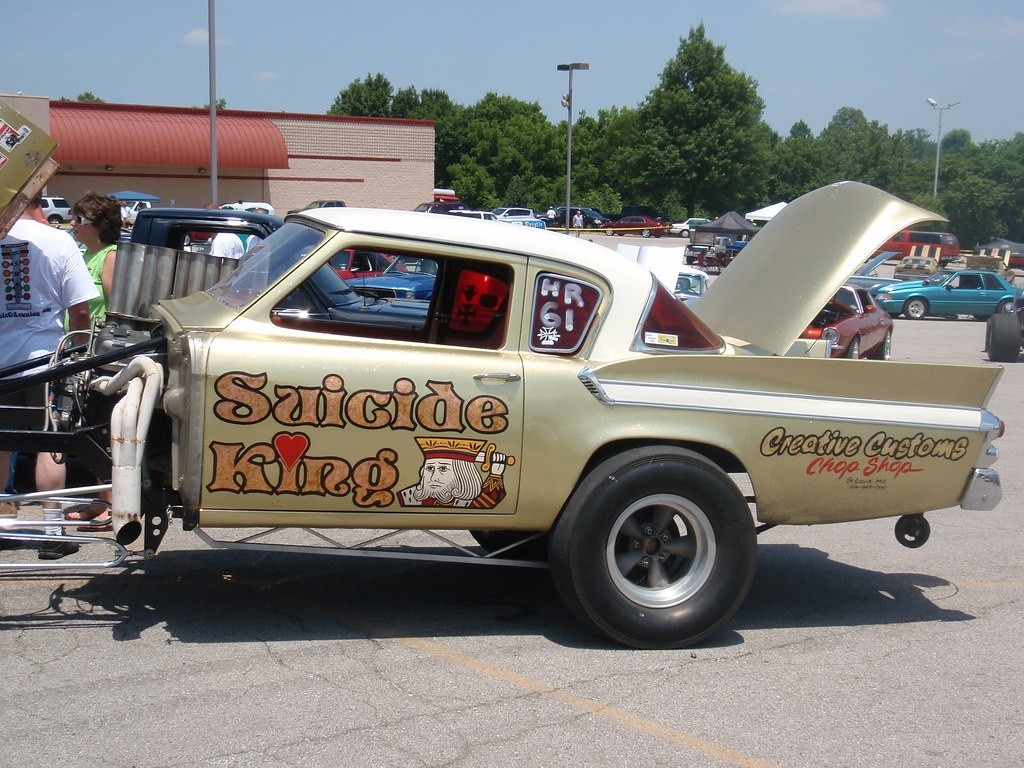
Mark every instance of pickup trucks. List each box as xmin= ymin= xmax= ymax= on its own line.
xmin=684 ymin=237 xmax=733 ymax=264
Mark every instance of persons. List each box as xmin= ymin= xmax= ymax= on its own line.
xmin=203 ymin=204 xmax=266 ymax=259
xmin=547 ymin=207 xmax=556 ymax=227
xmin=0 ymin=187 xmax=132 ymax=559
xmin=573 ymin=211 xmax=583 ymax=237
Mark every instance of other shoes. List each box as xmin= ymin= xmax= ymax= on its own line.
xmin=0 ymin=502 xmax=18 ymax=518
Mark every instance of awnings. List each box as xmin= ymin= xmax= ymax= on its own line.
xmin=49 ymin=100 xmax=289 ymax=171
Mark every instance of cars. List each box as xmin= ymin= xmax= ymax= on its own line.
xmin=537 ymin=206 xmax=715 ymax=237
xmin=873 ymin=256 xmax=1024 ymax=319
xmin=798 ymin=283 xmax=895 ymax=360
xmin=414 ymin=202 xmax=548 ymax=229
xmin=123 ymin=201 xmax=437 ymax=335
xmin=40 ymin=196 xmax=75 ymax=223
xmin=152 ymin=207 xmax=1010 ymax=649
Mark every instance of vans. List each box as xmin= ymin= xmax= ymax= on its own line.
xmin=879 ymin=230 xmax=961 ymax=260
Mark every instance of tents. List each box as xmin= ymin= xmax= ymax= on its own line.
xmin=108 ymin=190 xmax=160 ymax=203
xmin=694 ymin=212 xmax=760 ymax=258
xmin=745 ymin=202 xmax=788 ymax=221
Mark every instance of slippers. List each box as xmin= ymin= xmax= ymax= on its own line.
xmin=65 ymin=506 xmax=99 ymax=521
xmin=77 ymin=519 xmax=113 ymax=531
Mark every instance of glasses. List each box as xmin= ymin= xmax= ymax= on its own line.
xmin=72 ymin=214 xmax=109 ymax=225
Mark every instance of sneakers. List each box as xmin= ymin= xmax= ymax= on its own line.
xmin=39 ymin=531 xmax=79 ymax=559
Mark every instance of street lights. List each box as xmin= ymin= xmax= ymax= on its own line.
xmin=926 ymin=97 xmax=962 ymax=196
xmin=557 ymin=62 xmax=590 ymax=237
